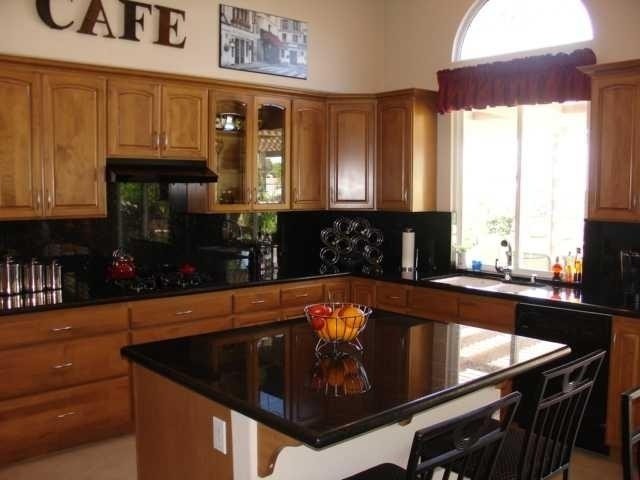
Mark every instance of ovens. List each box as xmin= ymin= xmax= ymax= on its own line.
xmin=505 ymin=302 xmax=614 ymax=456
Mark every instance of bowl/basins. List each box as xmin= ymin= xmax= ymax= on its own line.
xmin=216 ymin=111 xmax=243 ymax=132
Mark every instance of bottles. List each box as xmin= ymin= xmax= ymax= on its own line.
xmin=271 ymin=245 xmax=279 ymax=268
xmin=1 ymin=289 xmax=62 ymax=311
xmin=252 ymin=240 xmax=272 ymax=270
xmin=549 ymin=248 xmax=582 ymax=282
xmin=0 ymin=255 xmax=62 ymax=295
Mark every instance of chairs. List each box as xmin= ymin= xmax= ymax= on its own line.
xmin=341 ymin=391 xmax=522 ymax=480
xmin=619 ymin=385 xmax=640 ymax=480
xmin=438 ymin=350 xmax=607 ymax=480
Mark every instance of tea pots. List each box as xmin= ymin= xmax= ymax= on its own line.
xmin=108 ymin=247 xmax=135 ymax=282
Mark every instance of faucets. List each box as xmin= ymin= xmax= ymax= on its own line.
xmin=494 ymin=240 xmax=514 ymax=280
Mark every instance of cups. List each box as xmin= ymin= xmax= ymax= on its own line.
xmin=472 ymin=259 xmax=482 ymax=272
xmin=319 ymin=216 xmax=385 ymax=268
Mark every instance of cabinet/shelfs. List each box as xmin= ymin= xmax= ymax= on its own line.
xmin=576 ymin=59 xmax=640 ymax=224
xmin=351 ymin=277 xmax=376 ymax=308
xmin=458 ymin=293 xmax=518 ymax=335
xmin=108 ymin=67 xmax=209 ymax=161
xmin=291 ymin=88 xmax=328 ymax=211
xmin=605 ymin=316 xmax=640 ymax=463
xmin=376 ymin=282 xmax=408 ymax=315
xmin=130 ymin=291 xmax=234 ymax=345
xmin=376 ymin=87 xmax=438 ymax=212
xmin=0 ymin=301 xmax=133 ymax=469
xmin=328 ymin=93 xmax=376 ymax=211
xmin=281 ymin=277 xmax=323 ymax=321
xmin=323 ymin=275 xmax=351 ymax=305
xmin=234 ymin=281 xmax=281 ymax=329
xmin=0 ymin=55 xmax=108 ymax=220
xmin=208 ymin=79 xmax=291 ymax=212
xmin=408 ymin=285 xmax=458 ymax=324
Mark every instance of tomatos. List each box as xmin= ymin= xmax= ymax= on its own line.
xmin=323 ymin=306 xmax=332 ymax=318
xmin=308 ymin=305 xmax=327 ymax=316
xmin=310 ymin=316 xmax=325 ymax=330
xmin=311 ymin=378 xmax=325 ymax=392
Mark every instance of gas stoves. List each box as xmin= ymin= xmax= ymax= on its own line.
xmin=98 ymin=269 xmax=228 ymax=293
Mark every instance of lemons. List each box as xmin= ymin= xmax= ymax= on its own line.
xmin=324 ymin=359 xmax=366 ymax=394
xmin=319 ymin=315 xmax=345 ymax=341
xmin=332 ymin=307 xmax=340 ymax=317
xmin=338 ymin=306 xmax=366 ymax=329
xmin=342 ymin=325 xmax=357 ymax=342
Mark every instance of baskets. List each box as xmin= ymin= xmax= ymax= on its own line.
xmin=310 ymin=353 xmax=372 ymax=399
xmin=303 ymin=287 xmax=373 ymax=353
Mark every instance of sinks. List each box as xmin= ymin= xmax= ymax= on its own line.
xmin=492 ymin=283 xmax=537 ymax=294
xmin=431 ymin=275 xmax=501 ymax=290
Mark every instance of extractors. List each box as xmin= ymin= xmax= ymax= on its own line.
xmin=106 ymin=155 xmax=219 ymax=184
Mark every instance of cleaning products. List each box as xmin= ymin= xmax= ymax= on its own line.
xmin=551 ymin=248 xmax=582 ymax=282
